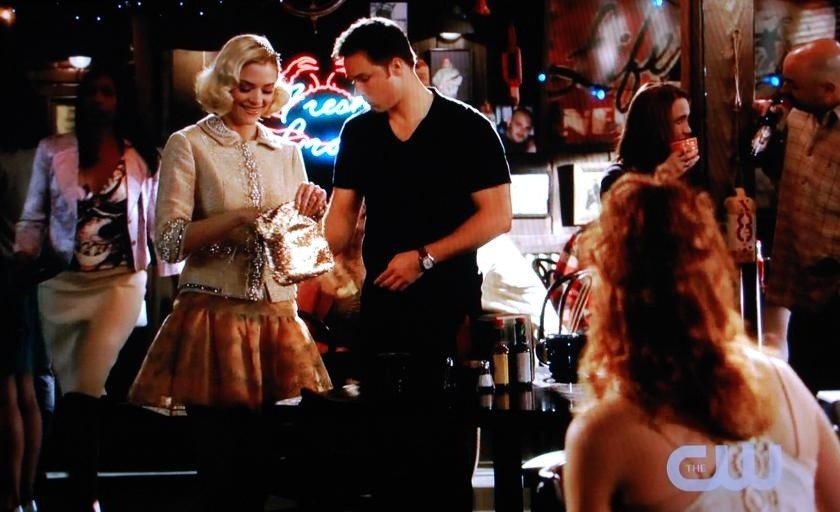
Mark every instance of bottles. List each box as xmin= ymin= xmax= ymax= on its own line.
xmin=491 ymin=315 xmax=512 ymax=393
xmin=720 ymin=185 xmax=758 ymax=267
xmin=512 ymin=314 xmax=532 ymax=392
xmin=474 ymin=359 xmax=496 ymax=396
xmin=743 ymin=102 xmax=779 ymax=169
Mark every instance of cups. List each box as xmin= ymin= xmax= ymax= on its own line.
xmin=534 ymin=332 xmax=577 ymax=385
xmin=669 ymin=137 xmax=699 ymax=165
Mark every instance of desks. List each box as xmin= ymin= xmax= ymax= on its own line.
xmin=474 ymin=384 xmax=571 ymax=512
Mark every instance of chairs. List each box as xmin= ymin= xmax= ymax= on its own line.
xmin=480 ymin=268 xmax=599 ymax=390
xmin=533 ymin=257 xmax=559 ymax=313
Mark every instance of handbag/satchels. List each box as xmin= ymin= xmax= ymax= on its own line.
xmin=253 ymin=198 xmax=336 ymax=287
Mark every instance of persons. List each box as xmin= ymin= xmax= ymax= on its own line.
xmin=1 ymin=0 xmax=840 ymax=512
